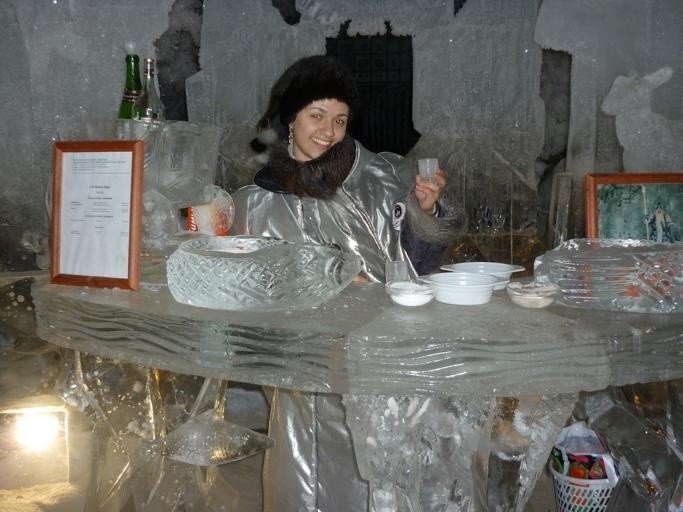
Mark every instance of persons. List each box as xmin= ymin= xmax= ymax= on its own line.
xmin=642 ymin=200 xmax=676 ymax=245
xmin=221 ymin=56 xmax=468 ymax=279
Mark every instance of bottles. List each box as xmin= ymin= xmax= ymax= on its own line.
xmin=119 ymin=54 xmax=166 ymax=119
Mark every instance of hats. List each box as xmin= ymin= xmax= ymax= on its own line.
xmin=250 ymin=57 xmax=363 ymax=166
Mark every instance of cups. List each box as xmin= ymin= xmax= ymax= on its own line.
xmin=418 ymin=159 xmax=439 ymax=184
xmin=384 ymin=261 xmax=409 ymax=286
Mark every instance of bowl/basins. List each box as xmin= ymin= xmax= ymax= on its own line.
xmin=418 ymin=262 xmax=525 ymax=309
xmin=506 ymin=282 xmax=558 ymax=310
xmin=387 ymin=281 xmax=434 ymax=306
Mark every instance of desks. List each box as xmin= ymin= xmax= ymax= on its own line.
xmin=28 ymin=254 xmax=682 ymax=511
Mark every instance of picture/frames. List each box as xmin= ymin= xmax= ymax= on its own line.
xmin=583 ymin=171 xmax=683 ymax=243
xmin=49 ymin=138 xmax=146 ymax=293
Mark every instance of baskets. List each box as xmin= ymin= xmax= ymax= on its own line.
xmin=550 ymin=454 xmax=616 ymax=511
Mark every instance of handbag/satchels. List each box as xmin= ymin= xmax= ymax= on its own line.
xmin=325 ymin=20 xmax=421 ymax=156
